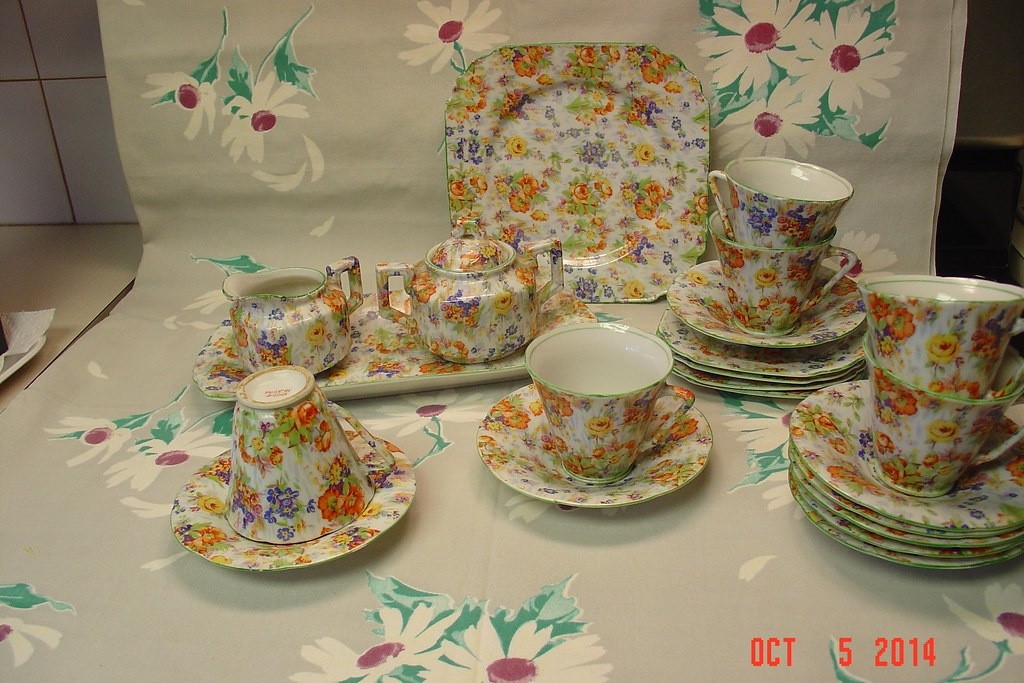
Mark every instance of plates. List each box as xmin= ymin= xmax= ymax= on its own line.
xmin=667 ymin=258 xmax=867 ymax=348
xmin=169 ymin=429 xmax=416 ymax=572
xmin=787 ymin=378 xmax=1023 ymax=571
xmin=443 ymin=41 xmax=712 ymax=305
xmin=476 ymin=382 xmax=715 ymax=507
xmin=192 ymin=279 xmax=597 ymax=402
xmin=653 ymin=306 xmax=869 ymax=398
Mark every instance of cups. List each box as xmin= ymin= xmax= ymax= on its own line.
xmin=221 ymin=364 xmax=397 ymax=546
xmin=523 ymin=321 xmax=696 ymax=483
xmin=708 ymin=157 xmax=855 ymax=247
xmin=708 ymin=208 xmax=858 ymax=337
xmin=859 ymin=331 xmax=1024 ymax=499
xmin=861 ymin=274 xmax=1024 ymax=397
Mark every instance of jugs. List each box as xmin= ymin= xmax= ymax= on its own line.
xmin=376 ymin=216 xmax=566 ymax=366
xmin=221 ymin=255 xmax=364 ymax=375
